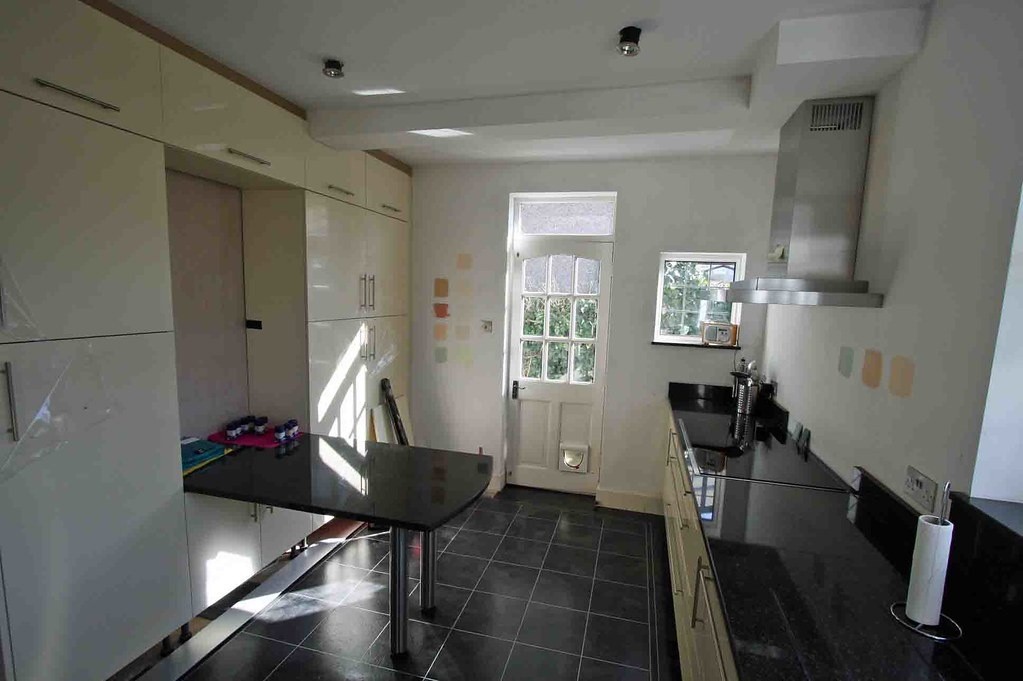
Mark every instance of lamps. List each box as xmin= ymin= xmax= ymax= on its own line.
xmin=322 ymin=58 xmax=344 ymax=79
xmin=615 ymin=25 xmax=642 ymax=57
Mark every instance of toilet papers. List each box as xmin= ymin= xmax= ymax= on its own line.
xmin=906 ymin=515 xmax=954 ymax=627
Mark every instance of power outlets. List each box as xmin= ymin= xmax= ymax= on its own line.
xmin=904 ymin=464 xmax=938 ymax=514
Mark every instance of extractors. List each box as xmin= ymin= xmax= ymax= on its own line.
xmin=726 ymin=96 xmax=884 ymax=308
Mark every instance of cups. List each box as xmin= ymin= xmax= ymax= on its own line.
xmin=734 ymin=413 xmax=756 ymax=444
xmin=737 ymin=383 xmax=758 ymax=416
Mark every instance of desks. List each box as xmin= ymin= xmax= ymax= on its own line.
xmin=182 ymin=425 xmax=494 ymax=656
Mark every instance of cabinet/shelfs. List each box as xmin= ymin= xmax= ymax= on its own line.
xmin=662 ymin=406 xmax=740 ymax=681
xmin=0 ymin=1 xmax=414 ymax=681
